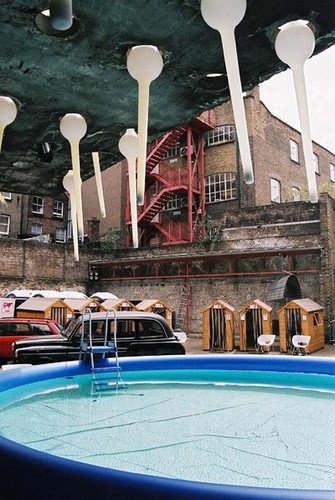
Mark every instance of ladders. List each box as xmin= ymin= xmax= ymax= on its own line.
xmin=79 ymin=307 xmax=129 ymax=397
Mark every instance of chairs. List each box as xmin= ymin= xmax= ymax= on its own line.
xmin=256 ymin=334 xmax=276 ymax=355
xmin=292 ymin=334 xmax=312 ymax=357
xmin=171 ymin=328 xmax=189 ymax=355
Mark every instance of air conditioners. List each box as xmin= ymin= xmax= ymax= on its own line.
xmin=181 ymin=196 xmax=194 ymax=208
xmin=179 ymin=145 xmax=195 ymax=159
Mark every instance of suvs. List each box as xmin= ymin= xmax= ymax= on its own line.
xmin=0 ymin=316 xmax=63 ymax=367
xmin=12 ymin=310 xmax=186 ymax=365
